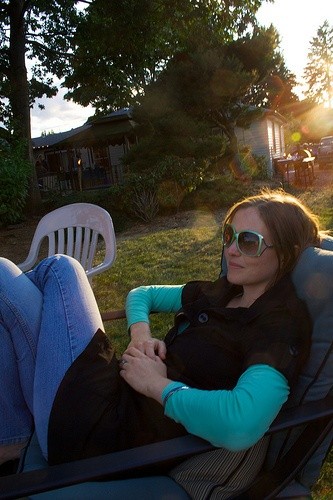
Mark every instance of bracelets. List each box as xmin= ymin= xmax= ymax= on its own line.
xmin=163 ymin=385 xmax=190 ymax=409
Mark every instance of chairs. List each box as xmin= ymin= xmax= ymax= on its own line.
xmin=0 ymin=235 xmax=333 ymax=500
xmin=16 ymin=202 xmax=116 ymax=286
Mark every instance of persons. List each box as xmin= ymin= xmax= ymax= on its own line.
xmin=35 ymin=161 xmax=46 ymax=186
xmin=0 ymin=190 xmax=318 ymax=470
xmin=293 ymin=142 xmax=316 ymax=167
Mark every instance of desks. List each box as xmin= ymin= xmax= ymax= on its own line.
xmin=277 ymin=157 xmax=316 ymax=187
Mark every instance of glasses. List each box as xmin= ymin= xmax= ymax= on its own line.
xmin=222 ymin=224 xmax=274 ymax=256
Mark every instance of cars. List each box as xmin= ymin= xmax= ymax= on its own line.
xmin=315 ymin=134 xmax=333 ymax=168
